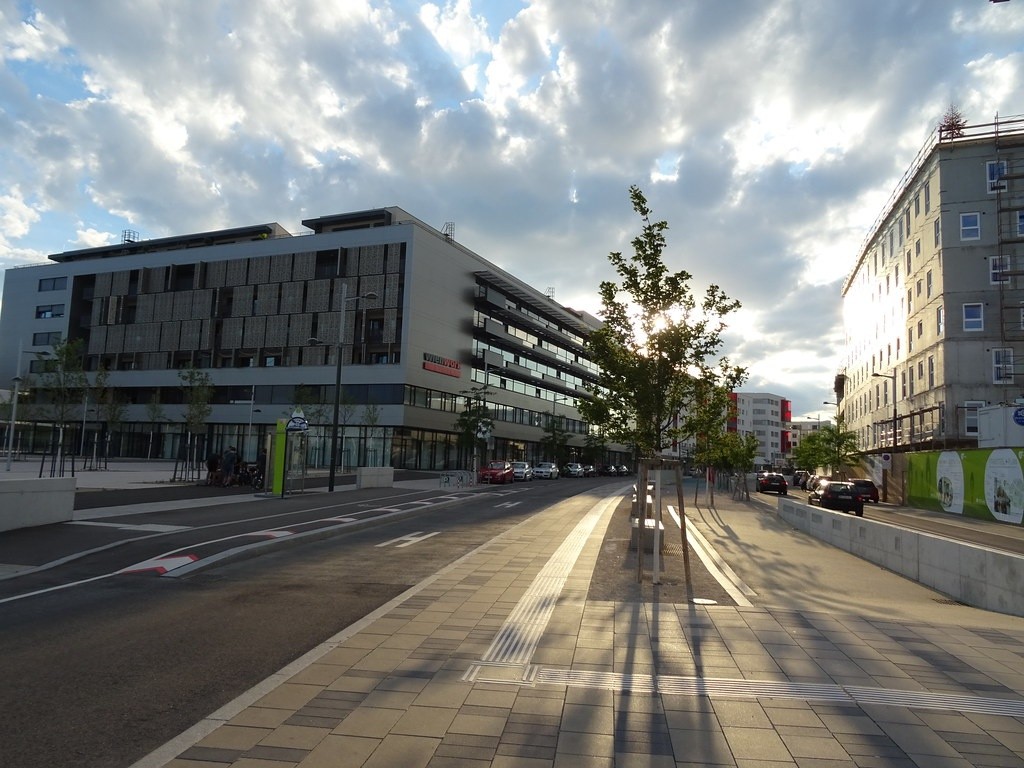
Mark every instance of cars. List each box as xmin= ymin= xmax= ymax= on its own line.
xmin=598 ymin=466 xmax=629 ymax=478
xmin=565 ymin=463 xmax=584 ymax=478
xmin=807 ymin=480 xmax=863 ymax=517
xmin=848 ymin=479 xmax=879 ymax=504
xmin=690 ymin=468 xmax=702 ymax=476
xmin=757 ymin=470 xmax=787 ymax=496
xmin=511 ymin=462 xmax=533 ymax=482
xmin=582 ymin=465 xmax=597 ymax=478
xmin=794 ymin=471 xmax=832 ymax=490
xmin=532 ymin=462 xmax=560 ymax=479
xmin=481 ymin=460 xmax=515 ymax=484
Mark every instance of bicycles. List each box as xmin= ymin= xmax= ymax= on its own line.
xmin=239 ymin=464 xmax=262 ymax=490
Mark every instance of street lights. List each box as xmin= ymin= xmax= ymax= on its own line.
xmin=244 ymin=389 xmax=261 ymax=467
xmin=871 ymin=372 xmax=897 ymax=452
xmin=552 ymin=398 xmax=569 ymax=422
xmin=307 ymin=294 xmax=380 ymax=492
xmin=791 ymin=418 xmax=820 ymax=450
xmin=80 ymin=409 xmax=95 ymax=456
xmin=6 ymin=351 xmax=52 ymax=470
xmin=824 ymin=402 xmax=839 ymax=458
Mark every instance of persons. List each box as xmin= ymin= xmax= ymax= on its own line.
xmin=207 ymin=445 xmax=266 ymax=487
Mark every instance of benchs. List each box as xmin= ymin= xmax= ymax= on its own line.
xmin=632 ymin=518 xmax=664 ymax=548
xmin=632 ymin=494 xmax=652 ymax=517
xmin=648 ymin=480 xmax=655 ymax=490
xmin=635 ymin=484 xmax=653 ymax=497
